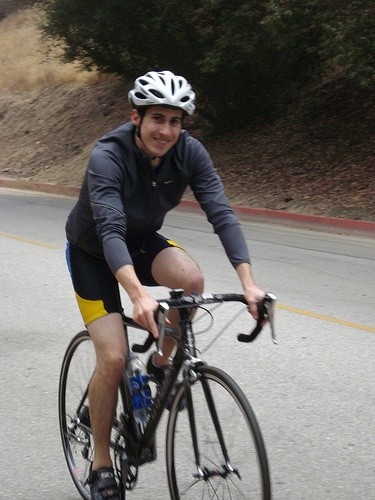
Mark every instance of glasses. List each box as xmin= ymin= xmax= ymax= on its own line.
xmin=150 ymin=173 xmax=176 ymax=190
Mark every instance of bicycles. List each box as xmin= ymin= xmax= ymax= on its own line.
xmin=58 ymin=288 xmax=279 ymax=500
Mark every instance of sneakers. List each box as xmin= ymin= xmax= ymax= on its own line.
xmin=147 ymin=352 xmax=186 ymax=411
xmin=87 ymin=461 xmax=121 ymax=500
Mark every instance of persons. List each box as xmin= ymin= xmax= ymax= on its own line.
xmin=65 ymin=70 xmax=270 ymax=500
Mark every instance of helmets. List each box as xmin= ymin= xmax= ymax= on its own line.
xmin=128 ymin=70 xmax=196 ymax=116
